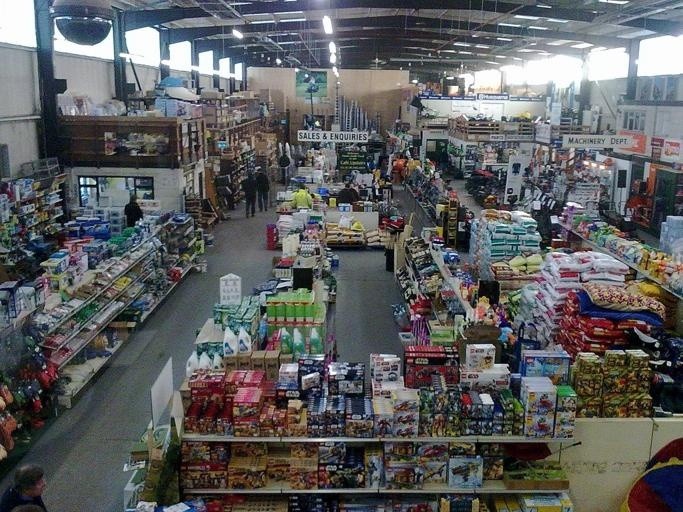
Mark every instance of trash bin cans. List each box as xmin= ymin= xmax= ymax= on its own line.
xmin=383 ymin=248 xmax=394 ymax=272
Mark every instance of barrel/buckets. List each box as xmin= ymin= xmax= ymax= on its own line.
xmin=212 ymin=350 xmax=226 ymax=370
xmin=223 ymin=325 xmax=238 ymax=355
xmin=281 ymin=326 xmax=322 ymax=361
xmin=238 ymin=327 xmax=252 ymax=353
xmin=185 ymin=350 xmax=199 ymax=378
xmin=199 ymin=349 xmax=212 ymax=370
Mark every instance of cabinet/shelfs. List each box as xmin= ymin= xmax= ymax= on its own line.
xmin=179 ymin=434 xmax=575 ymax=496
xmin=395 ymin=228 xmax=494 ymax=345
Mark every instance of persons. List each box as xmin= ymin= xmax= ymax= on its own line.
xmin=289 ymin=183 xmax=313 ymax=209
xmin=0 ymin=465 xmax=48 ymax=511
xmin=256 ymin=170 xmax=270 ymax=212
xmin=123 ymin=194 xmax=141 ymax=227
xmin=338 ymin=183 xmax=360 ymax=206
xmin=242 ymin=171 xmax=258 ymax=217
xmin=278 ymin=150 xmax=290 ymax=178
